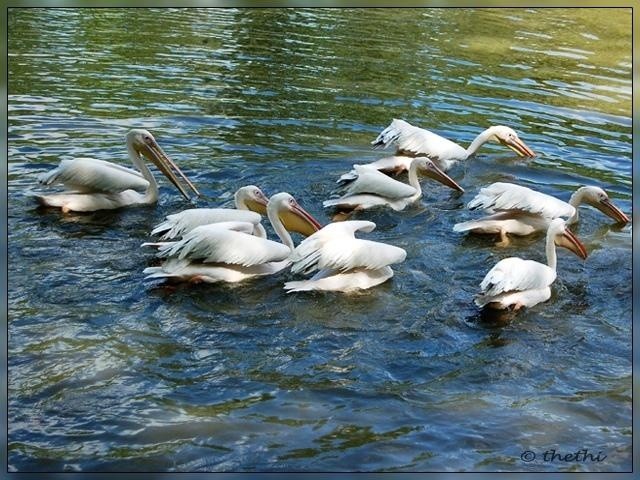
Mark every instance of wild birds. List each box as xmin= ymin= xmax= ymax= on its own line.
xmin=451 ymin=182 xmax=629 ymax=244
xmin=141 ymin=191 xmax=322 ymax=286
xmin=335 ymin=117 xmax=536 ymax=183
xmin=150 ymin=185 xmax=269 ymax=242
xmin=26 ymin=127 xmax=201 ymax=213
xmin=321 ymin=155 xmax=464 ymax=218
xmin=282 ymin=219 xmax=408 ymax=297
xmin=473 ymin=217 xmax=588 ymax=311
